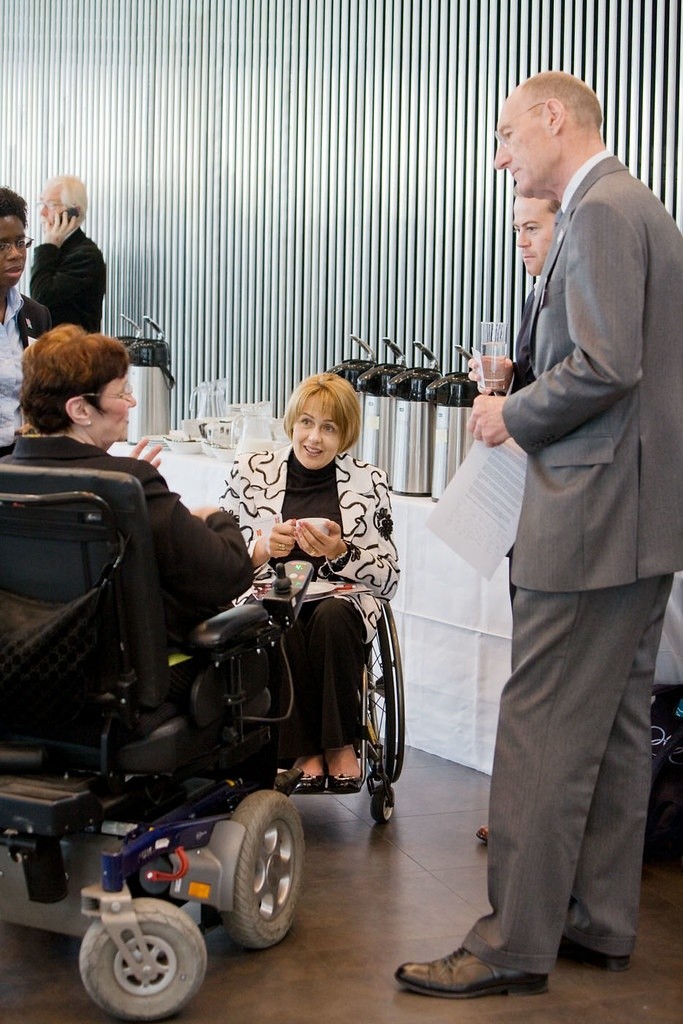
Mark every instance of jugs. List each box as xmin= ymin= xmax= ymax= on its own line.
xmin=231 ymin=402 xmax=275 ymax=459
xmin=190 ymin=377 xmax=228 ymax=418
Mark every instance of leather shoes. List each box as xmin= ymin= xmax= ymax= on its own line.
xmin=292 ymin=747 xmax=362 ymax=791
xmin=559 ymin=936 xmax=630 ymax=970
xmin=394 ymin=944 xmax=548 ymax=1000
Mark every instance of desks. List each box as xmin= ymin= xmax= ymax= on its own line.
xmin=112 ymin=440 xmax=682 ymax=778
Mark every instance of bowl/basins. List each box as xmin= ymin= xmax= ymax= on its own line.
xmin=200 ymin=441 xmax=215 ymax=457
xmin=211 ymin=449 xmax=235 ymax=460
xmin=162 ymin=436 xmax=205 ymax=454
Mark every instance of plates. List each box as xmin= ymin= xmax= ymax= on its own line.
xmin=305 ymin=581 xmax=336 ymax=599
xmin=142 ymin=435 xmax=168 ymax=447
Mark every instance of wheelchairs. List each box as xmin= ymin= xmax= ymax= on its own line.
xmin=233 ymin=556 xmax=407 ymax=823
xmin=0 ymin=464 xmax=304 ymax=1022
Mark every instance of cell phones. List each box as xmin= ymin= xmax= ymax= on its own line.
xmin=59 ymin=209 xmax=79 ymax=225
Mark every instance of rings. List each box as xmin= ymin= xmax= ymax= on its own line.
xmin=278 ymin=543 xmax=286 ymax=551
xmin=307 ymin=548 xmax=316 ymax=556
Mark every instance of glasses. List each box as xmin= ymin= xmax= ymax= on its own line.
xmin=0 ymin=236 xmax=34 ymax=252
xmin=37 ymin=202 xmax=76 ymax=210
xmin=80 ymin=381 xmax=134 ymax=399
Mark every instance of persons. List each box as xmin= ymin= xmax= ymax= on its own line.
xmin=0 ymin=325 xmax=253 ymax=737
xmin=0 ymin=175 xmax=105 ymax=458
xmin=216 ymin=373 xmax=400 ymax=796
xmin=395 ymin=71 xmax=683 ymax=998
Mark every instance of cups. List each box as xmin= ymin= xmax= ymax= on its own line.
xmin=480 ymin=322 xmax=507 ymax=389
xmin=296 ymin=518 xmax=331 ymax=536
